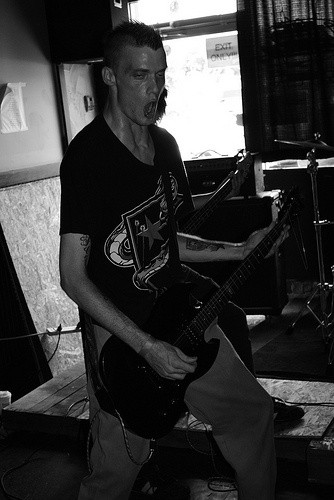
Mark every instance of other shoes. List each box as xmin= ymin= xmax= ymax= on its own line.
xmin=274 ymin=402 xmax=305 ymax=423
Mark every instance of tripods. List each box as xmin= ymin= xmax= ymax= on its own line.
xmin=288 ymin=152 xmax=334 ymax=331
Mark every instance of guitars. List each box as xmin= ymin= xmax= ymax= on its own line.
xmin=95 ymin=170 xmax=314 ymax=441
xmin=178 ymin=151 xmax=253 ymax=241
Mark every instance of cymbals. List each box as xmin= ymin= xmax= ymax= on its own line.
xmin=273 ymin=138 xmax=332 ymax=151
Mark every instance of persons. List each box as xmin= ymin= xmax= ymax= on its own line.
xmin=147 ymin=88 xmax=303 ymax=422
xmin=59 ymin=22 xmax=292 ymax=499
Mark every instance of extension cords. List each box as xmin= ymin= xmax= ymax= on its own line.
xmin=46 ymin=326 xmax=82 ymax=336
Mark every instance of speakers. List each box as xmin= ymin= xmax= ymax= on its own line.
xmin=184 ymin=153 xmax=265 ymax=197
xmin=179 ymin=192 xmax=289 ymax=315
xmin=261 ymin=157 xmax=334 ymax=282
